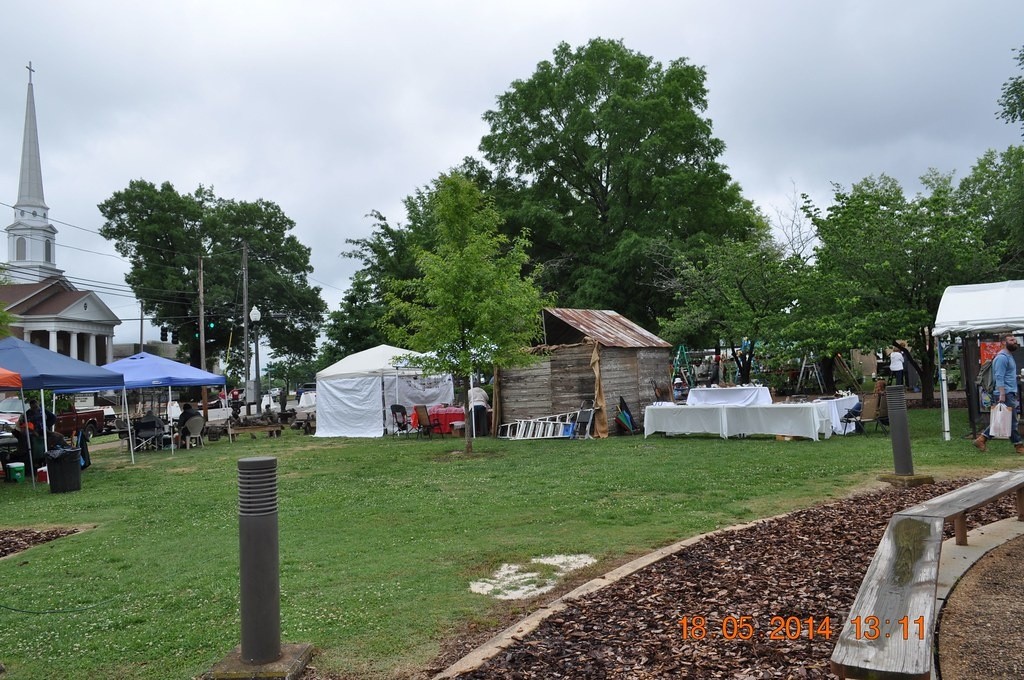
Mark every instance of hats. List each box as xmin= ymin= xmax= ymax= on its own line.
xmin=674 ymin=378 xmax=683 ymax=384
xmin=19 ymin=422 xmax=34 ymax=429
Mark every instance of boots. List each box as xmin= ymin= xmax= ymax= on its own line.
xmin=1016 ymin=445 xmax=1024 ymax=452
xmin=973 ymin=435 xmax=989 ymax=451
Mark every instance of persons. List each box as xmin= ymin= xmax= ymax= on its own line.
xmin=973 ymin=334 xmax=1024 ymax=452
xmin=297 ymin=387 xmax=304 ymax=404
xmin=178 ymin=404 xmax=204 ymax=448
xmin=674 ymin=378 xmax=683 ymax=399
xmin=229 ymin=387 xmax=239 ymax=401
xmin=138 ymin=410 xmax=164 ymax=450
xmin=890 ymin=343 xmax=909 ymax=387
xmin=818 ymin=358 xmax=837 ymax=394
xmin=15 ymin=400 xmax=57 ymax=469
xmin=840 ymin=380 xmax=886 ymax=433
xmin=280 ymin=387 xmax=287 ymax=412
xmin=468 ymin=382 xmax=489 ymax=436
xmin=219 ymin=388 xmax=228 ymax=411
xmin=711 ymin=362 xmax=727 ymax=385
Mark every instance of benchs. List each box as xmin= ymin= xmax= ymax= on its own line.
xmin=232 ymin=424 xmax=280 ymax=442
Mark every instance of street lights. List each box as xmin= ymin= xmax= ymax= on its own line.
xmin=249 ymin=306 xmax=264 ymax=419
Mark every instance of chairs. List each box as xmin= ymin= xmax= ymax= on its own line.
xmin=414 ymin=405 xmax=444 ymax=440
xmin=841 ymin=393 xmax=889 ymax=438
xmin=390 ymin=404 xmax=422 ymax=439
xmin=114 ymin=414 xmax=205 ymax=452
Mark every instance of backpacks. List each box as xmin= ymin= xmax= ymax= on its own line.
xmin=978 ymin=353 xmax=1010 ymax=394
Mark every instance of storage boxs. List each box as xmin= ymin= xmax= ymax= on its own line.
xmin=452 ymin=427 xmax=465 ymax=437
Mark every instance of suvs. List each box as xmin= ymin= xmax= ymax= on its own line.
xmin=229 ymin=388 xmax=264 ymax=404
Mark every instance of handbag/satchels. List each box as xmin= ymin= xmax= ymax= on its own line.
xmin=990 ymin=402 xmax=1013 ymax=438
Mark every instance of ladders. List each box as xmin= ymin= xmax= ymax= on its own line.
xmin=497 ymin=406 xmax=600 ymax=439
xmin=509 ymin=420 xmax=575 ymax=439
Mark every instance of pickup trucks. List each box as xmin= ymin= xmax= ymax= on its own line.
xmin=295 ymin=383 xmax=316 ymax=404
xmin=0 ymin=397 xmax=104 ymax=442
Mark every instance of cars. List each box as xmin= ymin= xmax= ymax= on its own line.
xmin=271 ymin=388 xmax=282 ymax=396
xmin=198 ymin=395 xmax=231 ymax=409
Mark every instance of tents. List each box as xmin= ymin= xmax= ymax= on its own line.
xmin=52 ymin=352 xmax=232 ymax=455
xmin=932 ymin=280 xmax=1024 ymax=441
xmin=0 ymin=367 xmax=35 ymax=486
xmin=0 ymin=336 xmax=135 ymax=483
xmin=313 ymin=344 xmax=454 ymax=437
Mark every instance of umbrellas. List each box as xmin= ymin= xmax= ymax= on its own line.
xmin=615 ymin=405 xmax=634 ymax=435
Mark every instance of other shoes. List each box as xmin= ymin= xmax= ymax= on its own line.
xmin=840 ymin=416 xmax=846 ymax=422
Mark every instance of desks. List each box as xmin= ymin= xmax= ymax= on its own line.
xmin=0 ymin=438 xmax=18 ymax=453
xmin=429 ymin=407 xmax=493 ymax=434
xmin=644 ymin=387 xmax=859 ymax=441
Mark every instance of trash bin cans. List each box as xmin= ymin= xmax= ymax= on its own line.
xmin=44 ymin=448 xmax=82 ymax=493
